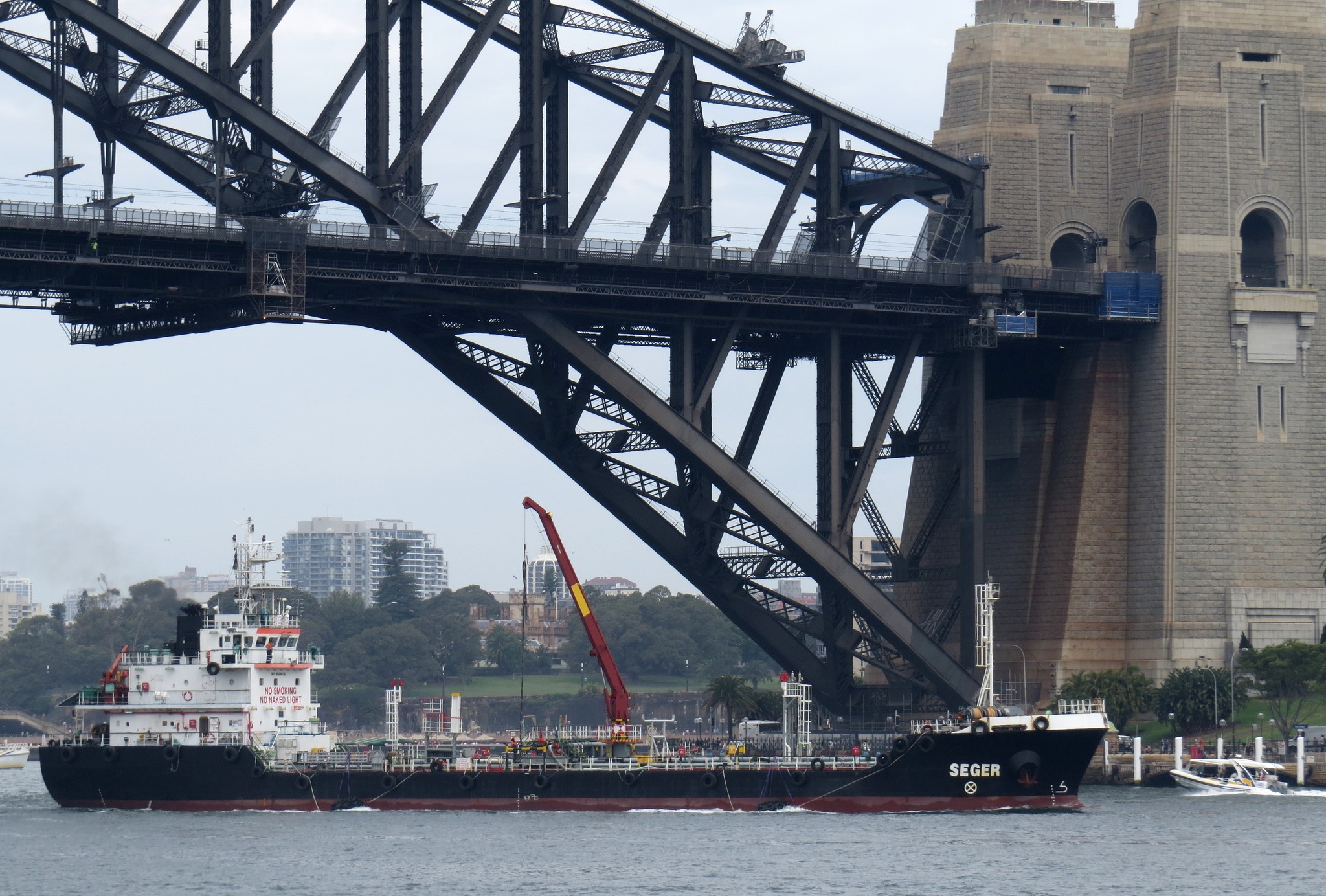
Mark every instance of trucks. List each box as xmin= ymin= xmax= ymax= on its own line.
xmin=738 ymin=720 xmax=783 ymax=751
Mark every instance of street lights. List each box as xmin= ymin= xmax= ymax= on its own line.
xmin=21 ymin=722 xmax=24 ymax=737
xmin=694 ymin=718 xmax=703 ymax=741
xmin=837 ymin=716 xmax=843 ymax=739
xmin=887 ymin=716 xmax=893 ymax=749
xmin=1267 ymin=719 xmax=1276 ymax=762
xmin=708 ymin=717 xmax=712 ymax=749
xmin=996 ymin=644 xmax=1027 ymax=714
xmin=1167 ymin=713 xmax=1176 ymax=754
xmin=1199 ymin=668 xmax=1218 ymax=759
xmin=63 ymin=721 xmax=66 ymax=732
xmin=743 ymin=717 xmax=749 ymax=750
xmin=720 ymin=717 xmax=725 ymax=748
xmin=732 ymin=718 xmax=737 ymax=740
xmin=1219 ymin=719 xmax=1226 ymax=739
xmin=1231 ymin=648 xmax=1249 ymax=754
xmin=1257 ymin=712 xmax=1264 ymax=737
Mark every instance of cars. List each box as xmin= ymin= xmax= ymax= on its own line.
xmin=1118 ymin=735 xmax=1133 ymax=752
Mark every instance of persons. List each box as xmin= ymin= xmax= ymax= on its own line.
xmin=682 ymin=728 xmax=719 ymax=736
xmin=1160 ymin=739 xmax=1169 ymax=753
xmin=495 ymin=729 xmax=510 ymax=736
xmin=345 ymin=730 xmax=363 ymax=737
xmin=1319 ymin=734 xmax=1325 ymax=752
xmin=266 ymin=639 xmax=273 ymax=663
xmin=234 ymin=635 xmax=239 ymax=646
xmin=1144 ymin=745 xmax=1151 ymax=754
xmin=258 ymin=642 xmax=264 ymax=647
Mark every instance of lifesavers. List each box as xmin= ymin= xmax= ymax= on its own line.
xmin=205 ymin=733 xmax=215 ymax=743
xmin=892 ymin=736 xmax=909 ymax=753
xmin=48 ymin=738 xmax=98 ymax=762
xmin=294 ymin=776 xmax=309 ymax=790
xmin=971 ymin=720 xmax=989 ymax=738
xmin=924 ymin=720 xmax=931 ymax=731
xmin=876 ymin=753 xmax=892 ymax=767
xmin=1033 ymin=716 xmax=1049 ymax=731
xmin=810 ymin=758 xmax=825 ymax=773
xmin=790 ymin=771 xmax=806 ymax=785
xmin=921 ymin=725 xmax=933 ymax=735
xmin=917 ymin=735 xmax=936 ymax=753
xmin=163 ymin=745 xmax=178 ymax=762
xmin=532 ymin=775 xmax=549 ymax=789
xmin=702 ymin=772 xmax=718 ymax=789
xmin=207 ymin=662 xmax=221 ymax=675
xmin=183 ymin=691 xmax=193 ymax=701
xmin=101 ymin=746 xmax=119 ymax=766
xmin=430 ymin=759 xmax=445 ymax=773
xmin=330 ymin=796 xmax=364 ymax=810
xmin=223 ymin=747 xmax=239 ymax=762
xmin=622 ymin=773 xmax=637 ymax=787
xmin=756 ymin=800 xmax=791 ymax=811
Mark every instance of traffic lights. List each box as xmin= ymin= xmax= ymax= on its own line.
xmin=895 ymin=715 xmax=901 ymax=726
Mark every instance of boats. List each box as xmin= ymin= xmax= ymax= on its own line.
xmin=1169 ymin=754 xmax=1288 ymax=795
xmin=0 ymin=739 xmax=31 ymax=769
xmin=40 ymin=517 xmax=1110 ymax=818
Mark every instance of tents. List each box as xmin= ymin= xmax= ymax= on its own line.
xmin=398 ymin=733 xmax=495 ymax=744
